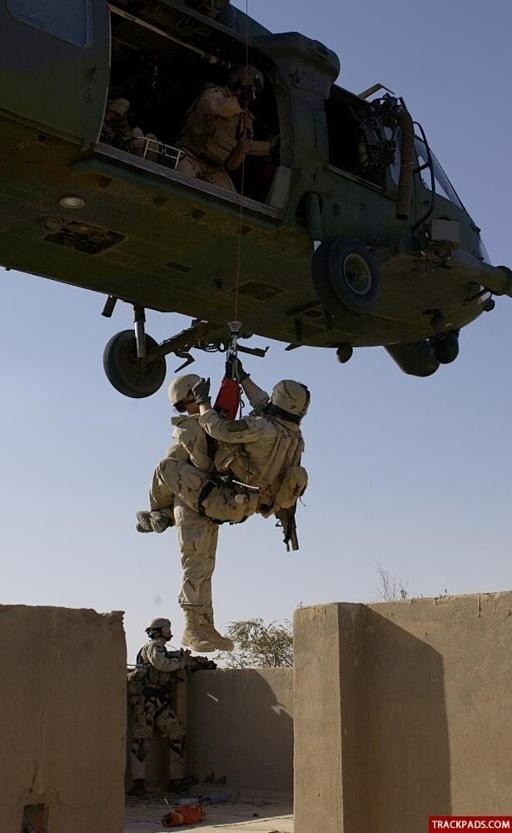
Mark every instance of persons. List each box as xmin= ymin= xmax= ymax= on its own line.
xmin=126 ymin=616 xmax=218 ymax=799
xmin=167 ymin=353 xmax=238 ymax=653
xmin=133 ymin=355 xmax=312 ymax=534
xmin=94 ymin=96 xmax=159 ymax=162
xmin=174 ymin=63 xmax=281 ymax=193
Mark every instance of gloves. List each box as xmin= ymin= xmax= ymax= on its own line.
xmin=226 ymin=353 xmax=251 ymax=384
xmin=191 ymin=377 xmax=211 ymax=405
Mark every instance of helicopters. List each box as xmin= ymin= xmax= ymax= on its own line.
xmin=0 ymin=0 xmax=510 ymax=397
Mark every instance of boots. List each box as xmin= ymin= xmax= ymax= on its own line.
xmin=181 ymin=605 xmax=235 ymax=653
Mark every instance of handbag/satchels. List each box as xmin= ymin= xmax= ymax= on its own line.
xmin=275 ymin=464 xmax=309 ymax=509
xmin=200 ymin=125 xmax=251 ymax=172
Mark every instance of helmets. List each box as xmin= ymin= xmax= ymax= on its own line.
xmin=167 ymin=373 xmax=201 ymax=407
xmin=271 ymin=378 xmax=312 ymax=418
xmin=145 ymin=618 xmax=174 ymax=641
xmin=230 ymin=63 xmax=265 ymax=90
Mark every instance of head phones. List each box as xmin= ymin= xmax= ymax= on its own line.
xmin=146 ymin=627 xmax=171 ymax=634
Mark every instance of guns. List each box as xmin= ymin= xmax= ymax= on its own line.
xmin=275 ymin=507 xmax=298 ymax=550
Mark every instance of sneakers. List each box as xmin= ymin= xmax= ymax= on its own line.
xmin=135 ymin=510 xmax=171 ymax=534
xmin=124 ymin=784 xmax=147 ymax=797
xmin=167 ymin=774 xmax=200 ymax=796
